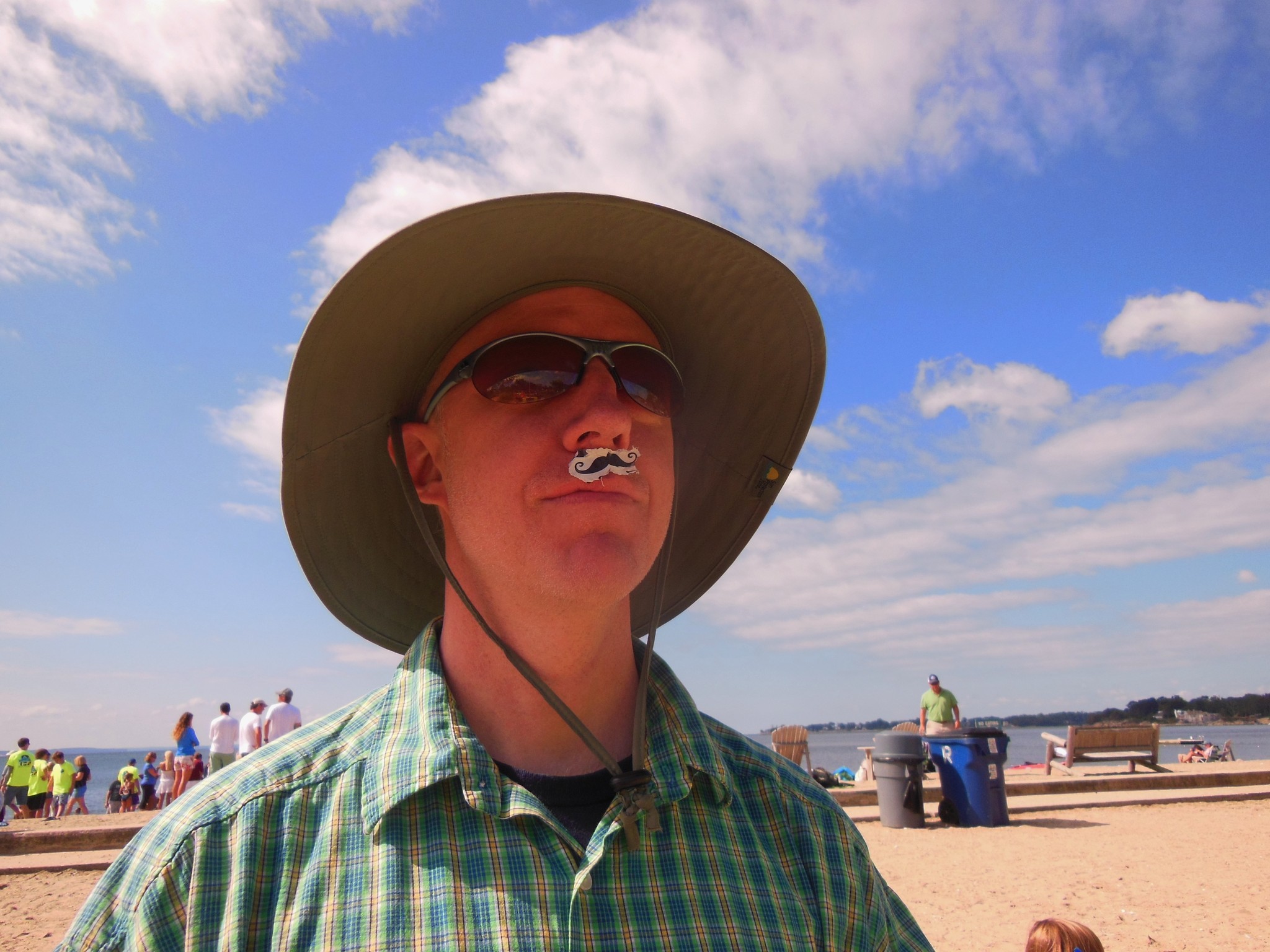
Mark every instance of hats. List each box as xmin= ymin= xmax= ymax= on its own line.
xmin=275 ymin=688 xmax=293 ymax=699
xmin=252 ymin=698 xmax=268 ymax=708
xmin=129 ymin=758 xmax=136 ymax=763
xmin=6 ymin=750 xmax=16 ymax=756
xmin=927 ymin=674 xmax=939 ymax=684
xmin=282 ymin=192 xmax=826 ymax=653
xmin=1202 ymin=740 xmax=1211 ymax=745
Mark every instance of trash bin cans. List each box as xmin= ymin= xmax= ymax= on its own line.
xmin=922 ymin=726 xmax=1011 ymax=828
xmin=872 ymin=729 xmax=924 ymax=827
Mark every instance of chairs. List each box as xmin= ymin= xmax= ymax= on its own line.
xmin=771 ymin=724 xmax=813 ymax=778
xmin=1192 ymin=738 xmax=1235 ymax=763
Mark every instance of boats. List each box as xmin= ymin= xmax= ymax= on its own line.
xmin=973 ymin=716 xmax=1011 ymax=740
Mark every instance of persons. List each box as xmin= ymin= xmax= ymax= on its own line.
xmin=185 ymin=753 xmax=210 ymax=792
xmin=1025 ymin=918 xmax=1104 ymax=952
xmin=236 ymin=698 xmax=269 ymax=761
xmin=172 ymin=712 xmax=199 ymax=802
xmin=51 ymin=193 xmax=938 ymax=952
xmin=263 ymin=687 xmax=302 ymax=745
xmin=1178 ymin=740 xmax=1216 ymax=764
xmin=0 ymin=737 xmax=91 ymax=827
xmin=918 ymin=675 xmax=960 ymax=737
xmin=105 ymin=751 xmax=174 ymax=815
xmin=208 ymin=703 xmax=240 ymax=776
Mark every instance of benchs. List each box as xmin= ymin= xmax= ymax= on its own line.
xmin=1040 ymin=721 xmax=1174 ymax=778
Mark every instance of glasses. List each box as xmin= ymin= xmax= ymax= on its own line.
xmin=423 ymin=331 xmax=687 ymax=426
